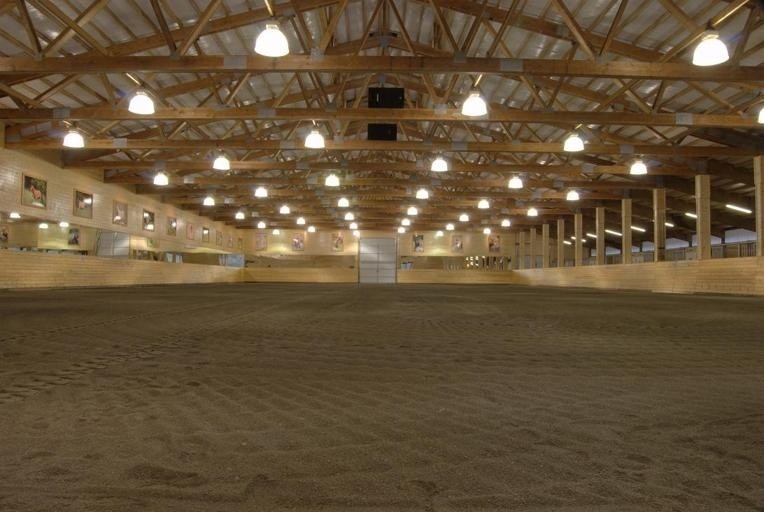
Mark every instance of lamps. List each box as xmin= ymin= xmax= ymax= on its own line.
xmin=304 ymin=124 xmax=325 ymax=149
xmin=693 ymin=19 xmax=729 ymax=66
xmin=416 ymin=186 xmax=430 ymax=200
xmin=254 ymin=18 xmax=290 ymax=57
xmin=629 ymin=155 xmax=648 ymax=177
xmin=213 ymin=207 xmax=729 ymax=247
xmin=154 ymin=170 xmax=169 ymax=186
xmin=566 ymin=188 xmax=580 ymax=200
xmin=213 ymin=153 xmax=230 ymax=171
xmin=338 ymin=197 xmax=349 ymax=206
xmin=62 ymin=122 xmax=85 ymax=148
xmin=431 ymin=155 xmax=448 ymax=172
xmin=462 ymin=84 xmax=487 ymax=118
xmin=564 ymin=125 xmax=585 ymax=153
xmin=128 ymin=88 xmax=155 ymax=115
xmin=254 ymin=187 xmax=268 ymax=199
xmin=203 ymin=196 xmax=216 ymax=208
xmin=326 ymin=172 xmax=340 ymax=186
xmin=508 ymin=172 xmax=523 ymax=189
xmin=478 ymin=197 xmax=491 ymax=210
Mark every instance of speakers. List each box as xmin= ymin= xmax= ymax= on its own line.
xmin=368 ymin=123 xmax=397 ymax=140
xmin=368 ymin=87 xmax=404 ymax=108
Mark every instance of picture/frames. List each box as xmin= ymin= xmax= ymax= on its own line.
xmin=112 ymin=199 xmax=129 ymax=226
xmin=21 ymin=172 xmax=49 ymax=211
xmin=142 ymin=208 xmax=155 ymax=232
xmin=202 ymin=227 xmax=210 ymax=243
xmin=72 ymin=188 xmax=93 ymax=219
xmin=186 ymin=221 xmax=194 ymax=240
xmin=166 ymin=216 xmax=177 ymax=237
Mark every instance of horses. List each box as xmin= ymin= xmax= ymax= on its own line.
xmin=29 ymin=185 xmax=41 ymax=200
